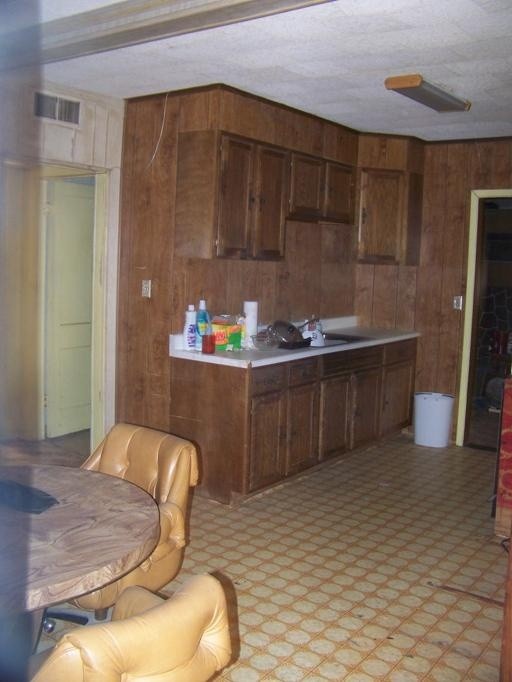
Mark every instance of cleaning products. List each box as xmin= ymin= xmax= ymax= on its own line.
xmin=184 ymin=299 xmax=243 ymax=352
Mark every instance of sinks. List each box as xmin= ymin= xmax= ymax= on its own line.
xmin=304 ymin=334 xmax=355 ymax=347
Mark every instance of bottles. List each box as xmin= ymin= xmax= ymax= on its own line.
xmin=183 ymin=299 xmax=212 ymax=352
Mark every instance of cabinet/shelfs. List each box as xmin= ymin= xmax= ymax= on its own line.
xmin=173 ymin=129 xmax=424 ymax=266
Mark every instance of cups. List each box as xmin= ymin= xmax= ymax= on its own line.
xmin=201 ymin=334 xmax=216 ymax=354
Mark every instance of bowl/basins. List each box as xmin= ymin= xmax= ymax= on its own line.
xmin=251 ymin=335 xmax=281 ymax=352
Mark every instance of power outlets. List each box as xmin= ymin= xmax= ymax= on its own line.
xmin=142 ymin=279 xmax=152 ymax=298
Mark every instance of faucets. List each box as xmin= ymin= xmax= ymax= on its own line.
xmin=296 ymin=317 xmax=320 ymax=331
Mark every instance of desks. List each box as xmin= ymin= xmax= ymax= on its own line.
xmin=1 ymin=463 xmax=159 ymax=655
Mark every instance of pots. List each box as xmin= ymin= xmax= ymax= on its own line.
xmin=266 ymin=315 xmax=320 ymax=348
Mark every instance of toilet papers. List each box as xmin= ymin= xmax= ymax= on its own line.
xmin=243 ymin=301 xmax=260 ymax=346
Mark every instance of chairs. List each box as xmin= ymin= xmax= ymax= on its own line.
xmin=65 ymin=422 xmax=200 ymax=621
xmin=26 ymin=572 xmax=233 ymax=682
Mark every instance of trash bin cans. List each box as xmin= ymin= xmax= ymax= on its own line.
xmin=414 ymin=391 xmax=456 ymax=448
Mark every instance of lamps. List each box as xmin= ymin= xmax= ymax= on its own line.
xmin=384 ymin=74 xmax=471 ymax=114
